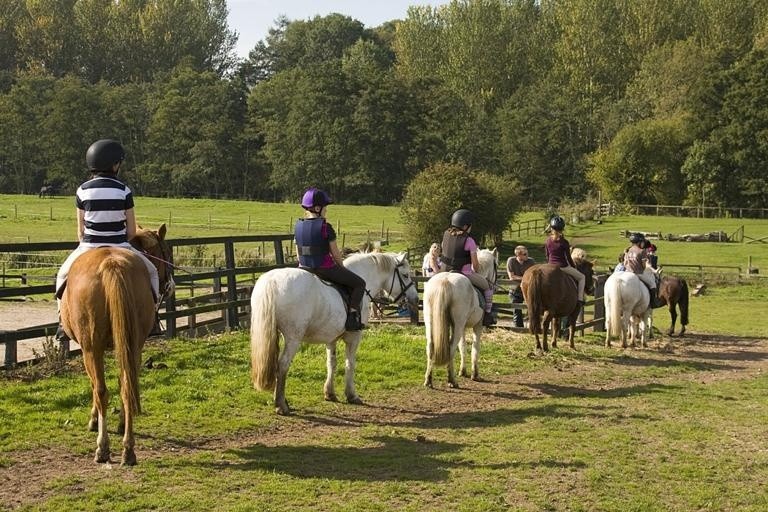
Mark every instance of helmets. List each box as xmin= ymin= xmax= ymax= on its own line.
xmin=451 ymin=209 xmax=473 ymax=228
xmin=86 ymin=140 xmax=125 ymax=172
xmin=630 ymin=233 xmax=656 ymax=251
xmin=302 ymin=189 xmax=333 ymax=208
xmin=551 ymin=217 xmax=565 ymax=231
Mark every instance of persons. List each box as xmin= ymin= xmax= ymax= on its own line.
xmin=544 ymin=216 xmax=587 ymax=305
xmin=440 ymin=208 xmax=498 ymax=326
xmin=422 ymin=241 xmax=441 ymax=276
xmin=613 ymin=232 xmax=661 ymax=309
xmin=293 ymin=187 xmax=368 ymax=332
xmin=53 ymin=135 xmax=162 ymax=343
xmin=506 ymin=245 xmax=537 ymax=327
xmin=562 ymin=247 xmax=593 ymax=342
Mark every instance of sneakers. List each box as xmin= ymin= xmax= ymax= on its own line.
xmin=347 ymin=312 xmax=364 ymax=329
xmin=56 ymin=327 xmax=70 ymax=341
xmin=484 ymin=312 xmax=496 ymax=325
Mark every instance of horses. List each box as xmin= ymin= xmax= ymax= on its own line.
xmin=603 ymin=261 xmax=663 ymax=349
xmin=423 ymin=247 xmax=499 ymax=388
xmin=60 ymin=222 xmax=176 ymax=467
xmin=520 ymin=257 xmax=598 ymax=356
xmin=39 ymin=185 xmax=54 ymax=199
xmin=249 ymin=248 xmax=420 ymax=416
xmin=608 ymin=266 xmax=689 ymax=336
